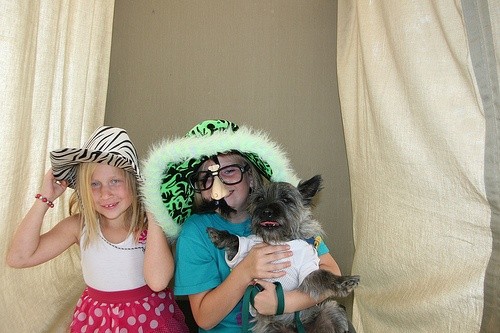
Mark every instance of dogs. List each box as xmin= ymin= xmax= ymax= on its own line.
xmin=207 ymin=164 xmax=360 ymax=333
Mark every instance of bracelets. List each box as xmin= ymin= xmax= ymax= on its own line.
xmin=35 ymin=193 xmax=55 ymax=208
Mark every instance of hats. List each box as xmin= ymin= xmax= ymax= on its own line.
xmin=50 ymin=126 xmax=146 ymax=189
xmin=135 ymin=119 xmax=302 ymax=246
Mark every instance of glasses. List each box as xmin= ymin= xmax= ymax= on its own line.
xmin=184 ymin=161 xmax=252 ymax=192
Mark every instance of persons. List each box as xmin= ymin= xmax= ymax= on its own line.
xmin=5 ymin=125 xmax=189 ymax=333
xmin=172 ymin=119 xmax=342 ymax=333
